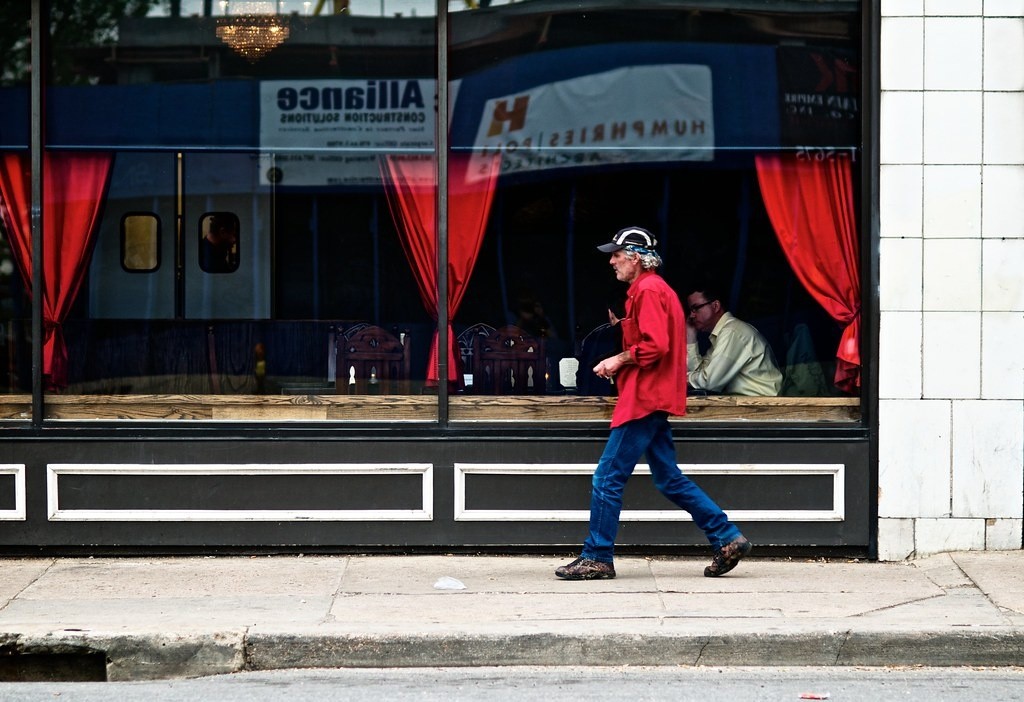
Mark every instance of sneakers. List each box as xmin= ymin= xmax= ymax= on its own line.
xmin=556 ymin=557 xmax=615 ymax=579
xmin=704 ymin=535 xmax=753 ymax=577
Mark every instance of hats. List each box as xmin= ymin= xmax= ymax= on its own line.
xmin=597 ymin=226 xmax=656 ymax=253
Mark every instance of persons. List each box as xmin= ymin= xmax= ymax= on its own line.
xmin=556 ymin=227 xmax=752 ymax=579
xmin=685 ymin=286 xmax=783 ymax=397
xmin=517 ymin=297 xmax=552 ymax=347
xmin=578 ymin=290 xmax=628 ymax=396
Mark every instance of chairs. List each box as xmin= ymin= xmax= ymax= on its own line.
xmin=334 ymin=321 xmax=817 ymax=396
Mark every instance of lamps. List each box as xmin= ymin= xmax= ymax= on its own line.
xmin=215 ymin=14 xmax=290 ymax=65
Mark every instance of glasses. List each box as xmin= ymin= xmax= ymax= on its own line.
xmin=687 ymin=300 xmax=712 ymax=313
